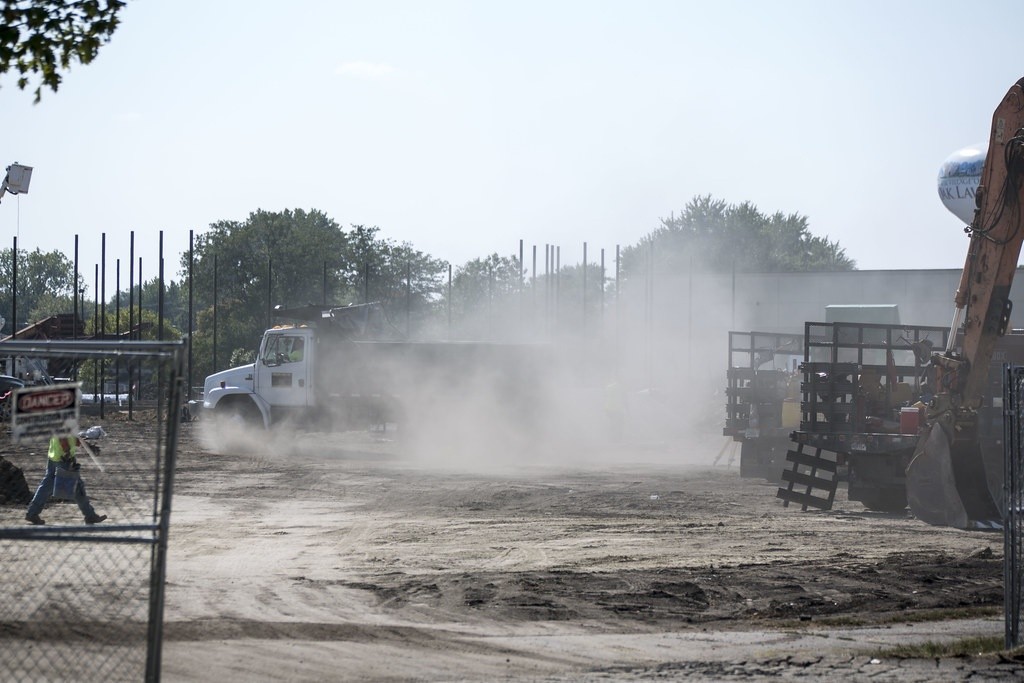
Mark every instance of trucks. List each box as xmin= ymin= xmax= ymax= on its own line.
xmin=202 ymin=326 xmax=597 ymax=436
xmin=725 ymin=330 xmax=824 ymax=482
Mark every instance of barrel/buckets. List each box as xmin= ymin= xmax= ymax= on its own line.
xmin=901 ymin=407 xmax=918 ymax=434
xmin=51 ymin=466 xmax=80 ymax=500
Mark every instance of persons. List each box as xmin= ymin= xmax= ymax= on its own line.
xmin=25 ymin=436 xmax=107 ymax=525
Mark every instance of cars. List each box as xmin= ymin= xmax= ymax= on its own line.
xmin=1 ymin=375 xmax=32 ymax=420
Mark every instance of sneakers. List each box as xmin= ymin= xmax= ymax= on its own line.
xmin=24 ymin=513 xmax=45 ymax=525
xmin=84 ymin=514 xmax=107 ymax=525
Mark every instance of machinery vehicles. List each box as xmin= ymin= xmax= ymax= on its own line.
xmin=789 ymin=71 xmax=1024 ymax=524
